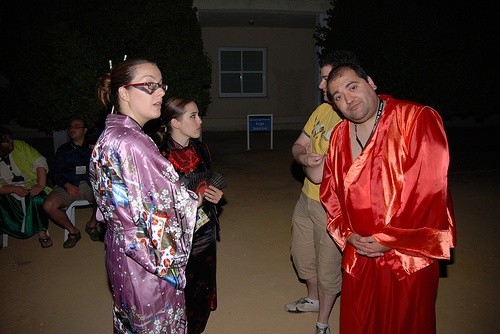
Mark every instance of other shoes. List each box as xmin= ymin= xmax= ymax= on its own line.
xmin=39 ymin=237 xmax=53 ymax=248
xmin=85 ymin=222 xmax=102 ymax=241
xmin=63 ymin=229 xmax=81 ymax=248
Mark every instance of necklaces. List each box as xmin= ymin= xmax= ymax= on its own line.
xmin=70 ymin=143 xmax=76 ymax=149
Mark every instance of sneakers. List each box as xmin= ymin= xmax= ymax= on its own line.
xmin=285 ymin=297 xmax=320 ymax=312
xmin=316 ymin=322 xmax=330 ymax=334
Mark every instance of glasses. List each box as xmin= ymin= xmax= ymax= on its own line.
xmin=69 ymin=125 xmax=83 ymax=129
xmin=124 ymin=82 xmax=168 ymax=92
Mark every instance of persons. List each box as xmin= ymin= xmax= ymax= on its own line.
xmin=43 ymin=118 xmax=100 ymax=248
xmin=0 ymin=126 xmax=53 ymax=248
xmin=159 ymin=97 xmax=224 ymax=334
xmin=284 ymin=55 xmax=358 ymax=333
xmin=320 ymin=62 xmax=456 ymax=334
xmin=88 ymin=52 xmax=203 ymax=334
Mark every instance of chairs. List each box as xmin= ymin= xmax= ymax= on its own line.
xmin=65 ymin=200 xmax=93 ymax=242
